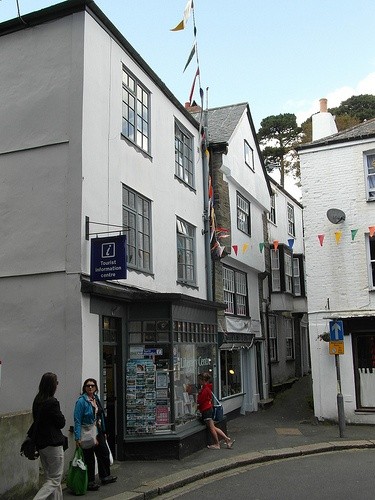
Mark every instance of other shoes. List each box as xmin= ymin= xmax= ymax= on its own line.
xmin=88 ymin=484 xmax=99 ymax=491
xmin=100 ymin=475 xmax=118 ymax=484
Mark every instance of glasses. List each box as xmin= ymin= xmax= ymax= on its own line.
xmin=86 ymin=384 xmax=95 ymax=388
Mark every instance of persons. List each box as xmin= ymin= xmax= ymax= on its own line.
xmin=73 ymin=378 xmax=117 ymax=490
xmin=197 ymin=371 xmax=235 ymax=450
xmin=31 ymin=373 xmax=66 ymax=500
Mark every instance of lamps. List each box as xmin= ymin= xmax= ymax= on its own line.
xmin=327 ymin=209 xmax=346 ymax=224
xmin=228 ymin=369 xmax=239 ymax=388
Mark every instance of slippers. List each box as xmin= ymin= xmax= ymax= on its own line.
xmin=227 ymin=439 xmax=236 ymax=449
xmin=207 ymin=444 xmax=220 ymax=450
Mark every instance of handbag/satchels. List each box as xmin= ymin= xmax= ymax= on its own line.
xmin=67 ymin=442 xmax=89 ymax=496
xmin=78 ymin=422 xmax=97 ymax=449
xmin=19 ymin=436 xmax=39 ymax=461
xmin=212 ymin=403 xmax=224 ymax=422
